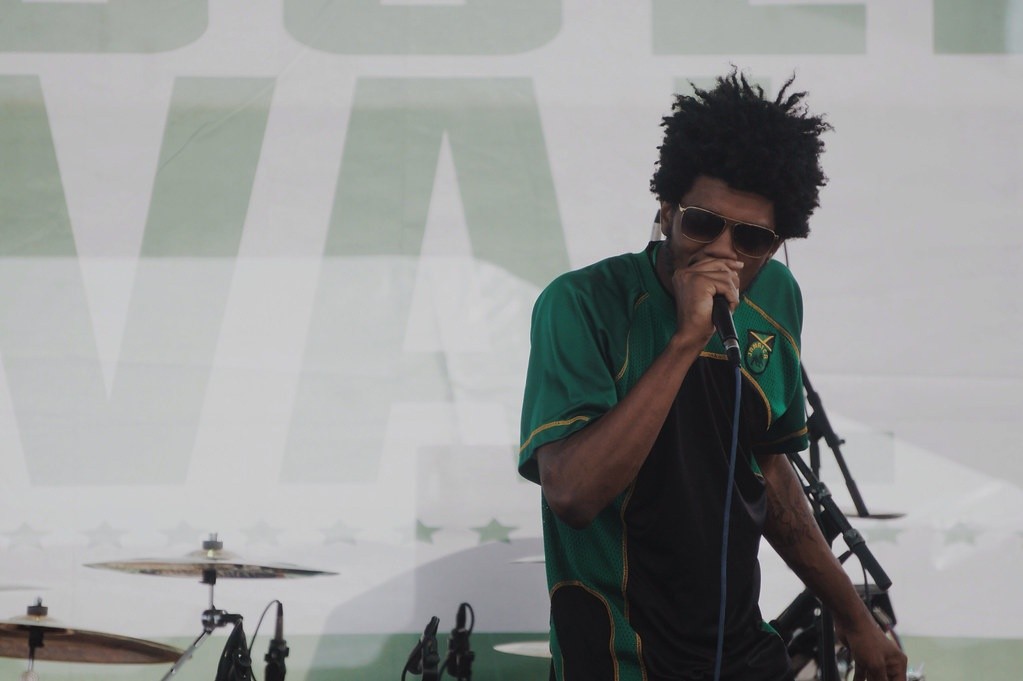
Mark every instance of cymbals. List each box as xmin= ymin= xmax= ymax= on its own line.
xmin=77 ymin=546 xmax=347 ymax=586
xmin=0 ymin=613 xmax=194 ymax=666
xmin=492 ymin=639 xmax=552 ymax=660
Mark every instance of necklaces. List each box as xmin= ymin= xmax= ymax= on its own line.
xmin=653 ymin=241 xmax=662 ymax=267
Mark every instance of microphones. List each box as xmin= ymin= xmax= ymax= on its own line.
xmin=406 ymin=616 xmax=439 ymax=674
xmin=712 ymin=293 xmax=740 ymax=367
xmin=446 ymin=602 xmax=466 ymax=677
xmin=264 ymin=603 xmax=285 ymax=681
xmin=767 ymin=549 xmax=852 ymax=642
xmin=214 ymin=618 xmax=247 ymax=681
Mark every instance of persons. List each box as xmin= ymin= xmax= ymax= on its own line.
xmin=518 ymin=74 xmax=908 ymax=681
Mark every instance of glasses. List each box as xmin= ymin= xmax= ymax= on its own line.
xmin=671 ymin=201 xmax=781 ymax=259
